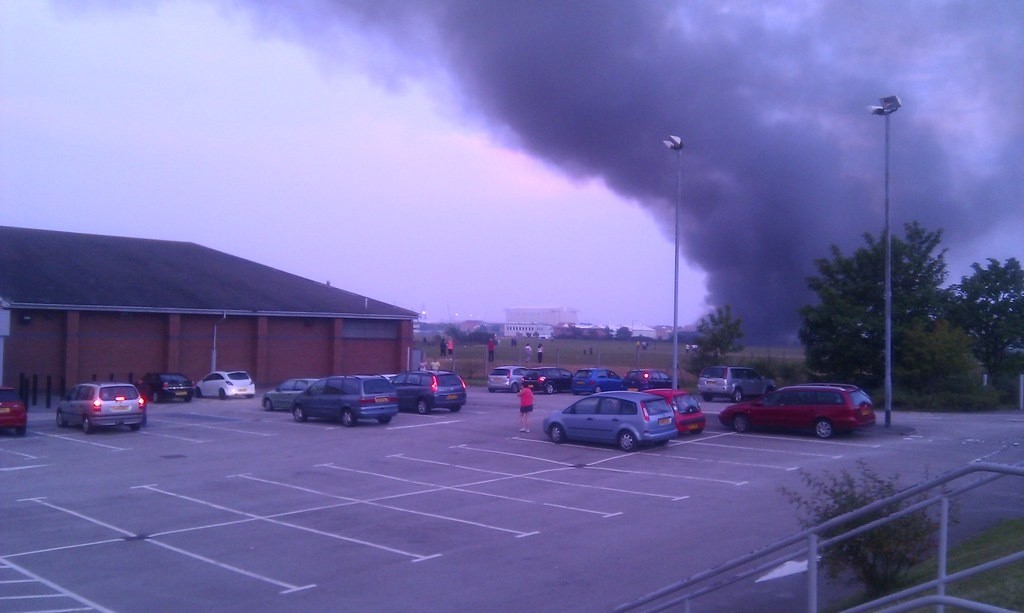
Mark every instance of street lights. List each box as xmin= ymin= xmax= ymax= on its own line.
xmin=661 ymin=134 xmax=687 ymax=388
xmin=866 ymin=93 xmax=902 ymax=429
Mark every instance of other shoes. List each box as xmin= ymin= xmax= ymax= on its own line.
xmin=525 ymin=429 xmax=530 ymax=432
xmin=520 ymin=428 xmax=525 ymax=431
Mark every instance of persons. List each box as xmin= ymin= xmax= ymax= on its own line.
xmin=511 ymin=338 xmax=513 ymax=345
xmin=418 ymin=361 xmax=430 ymax=370
xmin=440 ymin=339 xmax=446 ymax=357
xmin=589 ymin=344 xmax=592 ymax=354
xmin=583 ymin=344 xmax=586 ymax=354
xmin=431 ymin=359 xmax=440 ymax=370
xmin=447 ymin=338 xmax=454 ymax=361
xmin=518 ymin=382 xmax=531 ymax=433
xmin=685 ymin=343 xmax=699 ymax=353
xmin=514 ymin=340 xmax=517 ymax=345
xmin=537 ymin=343 xmax=544 ymax=362
xmin=635 ymin=339 xmax=649 ymax=350
xmin=524 ymin=343 xmax=532 ymax=362
xmin=488 ymin=338 xmax=496 ymax=362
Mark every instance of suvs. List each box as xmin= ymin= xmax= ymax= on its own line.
xmin=696 ymin=366 xmax=777 ymax=402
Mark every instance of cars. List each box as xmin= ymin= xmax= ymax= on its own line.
xmin=289 ymin=373 xmax=401 ymax=428
xmin=486 ymin=365 xmax=529 ymax=393
xmin=621 ymin=369 xmax=679 ymax=390
xmin=543 ymin=391 xmax=681 ymax=453
xmin=138 ymin=372 xmax=195 ymax=402
xmin=196 ymin=370 xmax=257 ymax=399
xmin=522 ymin=367 xmax=573 ymax=393
xmin=261 ymin=377 xmax=318 ymax=412
xmin=642 ymin=389 xmax=706 ymax=434
xmin=718 ymin=384 xmax=878 ymax=440
xmin=0 ymin=385 xmax=27 ymax=434
xmin=570 ymin=368 xmax=627 ymax=392
xmin=56 ymin=381 xmax=147 ymax=431
xmin=390 ymin=370 xmax=466 ymax=415
